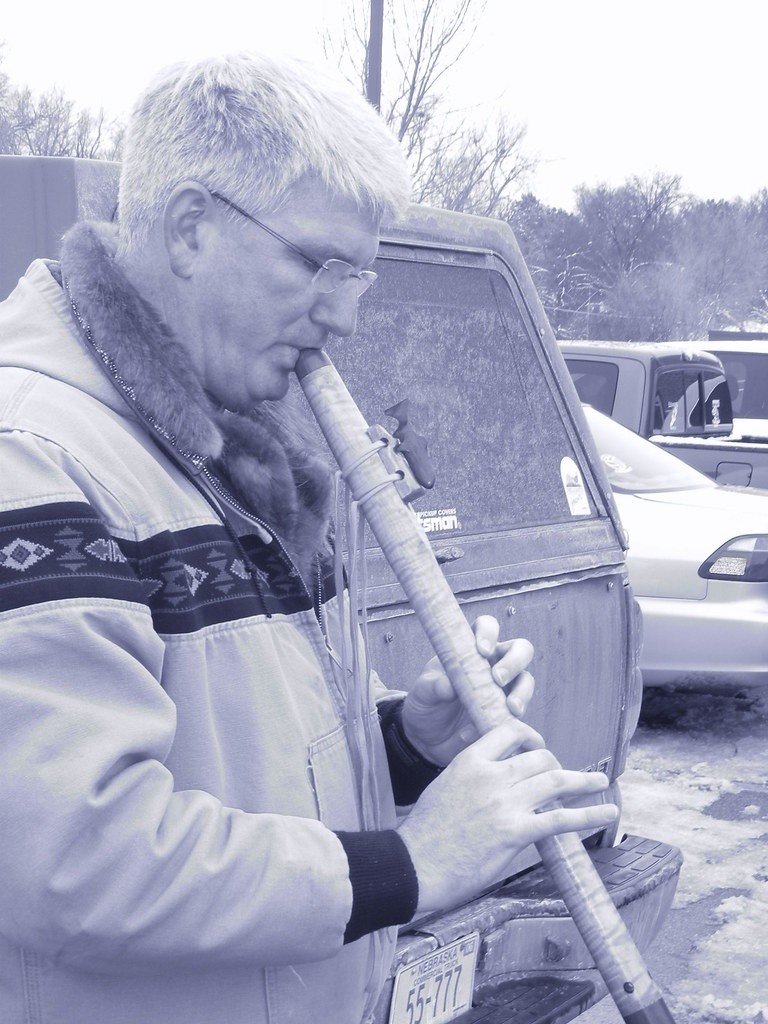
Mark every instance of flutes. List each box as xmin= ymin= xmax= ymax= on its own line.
xmin=294 ymin=346 xmax=673 ymax=1024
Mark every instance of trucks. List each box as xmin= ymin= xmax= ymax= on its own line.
xmin=557 ymin=340 xmax=768 ymax=491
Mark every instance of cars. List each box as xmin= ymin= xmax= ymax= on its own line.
xmin=699 ymin=341 xmax=767 ymax=441
xmin=0 ymin=156 xmax=683 ymax=1024
xmin=581 ymin=401 xmax=768 ymax=689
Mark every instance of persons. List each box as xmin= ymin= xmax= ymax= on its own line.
xmin=0 ymin=48 xmax=618 ymax=1024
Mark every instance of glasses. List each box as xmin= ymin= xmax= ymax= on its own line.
xmin=209 ymin=190 xmax=378 ymax=297
